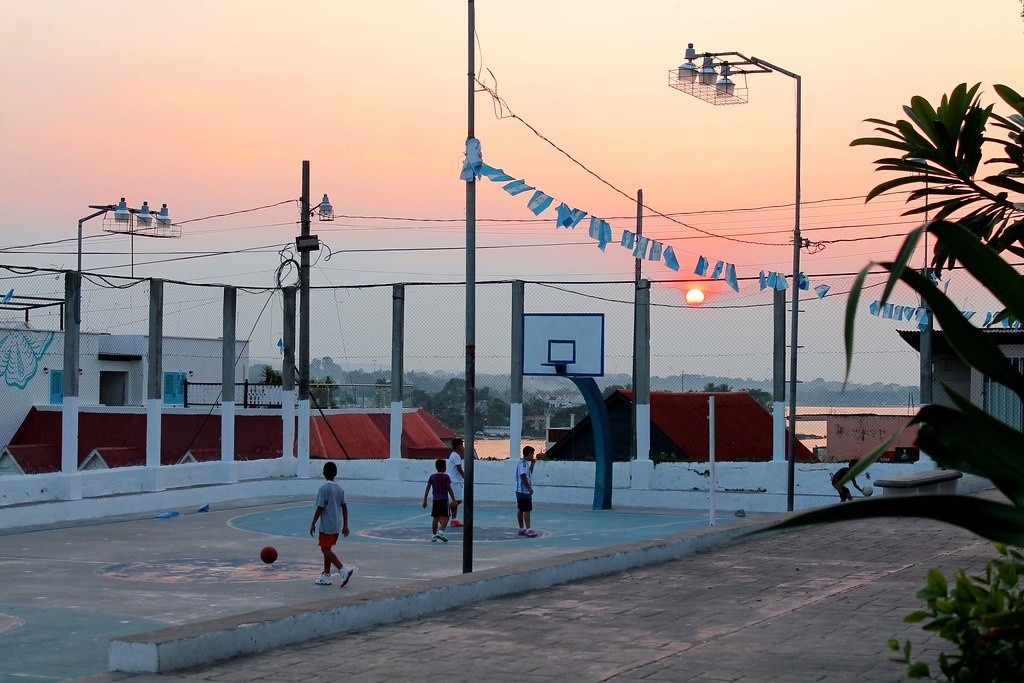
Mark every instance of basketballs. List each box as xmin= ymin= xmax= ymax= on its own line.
xmin=862 ymin=486 xmax=873 ymax=496
xmin=260 ymin=546 xmax=278 ymax=564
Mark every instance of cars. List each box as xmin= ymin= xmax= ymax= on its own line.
xmin=896 ymin=446 xmax=919 ymax=464
xmin=876 ymin=450 xmax=895 ymax=463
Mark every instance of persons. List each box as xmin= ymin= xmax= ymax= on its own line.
xmin=515 ymin=446 xmax=538 ymax=537
xmin=423 ymin=437 xmax=464 ymax=542
xmin=310 ymin=462 xmax=353 ymax=587
xmin=832 ymin=459 xmax=864 ymax=502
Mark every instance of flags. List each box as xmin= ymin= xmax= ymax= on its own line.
xmin=460 ymin=139 xmax=1024 ymax=333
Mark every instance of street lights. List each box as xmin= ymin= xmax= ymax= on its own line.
xmin=668 ymin=43 xmax=802 ymax=511
xmin=296 ymin=161 xmax=334 ymax=479
xmin=61 ymin=198 xmax=181 ymax=472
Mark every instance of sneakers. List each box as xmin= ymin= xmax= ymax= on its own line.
xmin=431 ymin=535 xmax=437 ymax=542
xmin=450 ymin=520 xmax=463 ymax=527
xmin=439 ymin=517 xmax=444 ymax=524
xmin=436 ymin=532 xmax=448 ymax=542
xmin=525 ymin=530 xmax=537 ymax=536
xmin=314 ymin=571 xmax=332 ymax=585
xmin=339 ymin=567 xmax=353 ymax=587
xmin=518 ymin=529 xmax=526 ymax=536
